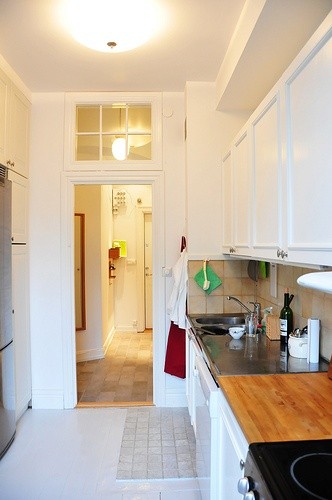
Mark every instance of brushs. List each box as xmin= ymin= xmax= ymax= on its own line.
xmin=202 ymin=257 xmax=210 ymax=291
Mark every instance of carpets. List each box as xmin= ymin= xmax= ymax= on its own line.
xmin=116 ymin=407 xmax=197 ymax=480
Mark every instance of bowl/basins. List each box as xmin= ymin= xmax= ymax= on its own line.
xmin=229 ymin=340 xmax=245 ymax=350
xmin=228 ymin=327 xmax=246 ymax=340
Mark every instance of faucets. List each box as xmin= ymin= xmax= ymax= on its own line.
xmin=227 ymin=296 xmax=261 ymax=325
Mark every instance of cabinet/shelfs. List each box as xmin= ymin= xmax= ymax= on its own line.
xmin=252 ymin=8 xmax=332 ymax=270
xmin=219 ymin=390 xmax=249 ymax=500
xmin=8 ymin=169 xmax=29 ymax=245
xmin=1 ymin=71 xmax=32 ymax=183
xmin=186 ymin=324 xmax=196 ymax=426
xmin=10 ymin=244 xmax=32 ymax=414
xmin=223 ymin=116 xmax=252 ymax=259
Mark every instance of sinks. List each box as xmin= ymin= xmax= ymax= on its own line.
xmin=196 ymin=316 xmax=252 ymax=324
xmin=201 ymin=325 xmax=258 ymax=336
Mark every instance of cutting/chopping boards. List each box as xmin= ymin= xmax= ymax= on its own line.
xmin=216 ymin=372 xmax=332 ymax=444
xmin=270 ymin=262 xmax=278 ymax=299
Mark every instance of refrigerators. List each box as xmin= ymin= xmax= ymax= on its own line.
xmin=0 ymin=176 xmax=16 ymax=459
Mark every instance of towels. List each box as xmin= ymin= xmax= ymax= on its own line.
xmin=169 ymin=250 xmax=189 ymax=330
xmin=164 ymin=236 xmax=187 ymax=378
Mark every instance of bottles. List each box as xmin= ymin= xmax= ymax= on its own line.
xmin=247 ymin=313 xmax=255 ymax=336
xmin=256 ymin=324 xmax=263 ymax=340
xmin=279 ymin=287 xmax=293 ymax=351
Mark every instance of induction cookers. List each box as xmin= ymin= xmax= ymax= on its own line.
xmin=238 ymin=438 xmax=332 ymax=500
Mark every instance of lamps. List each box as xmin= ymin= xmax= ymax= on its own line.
xmin=112 ymin=109 xmax=129 ymax=159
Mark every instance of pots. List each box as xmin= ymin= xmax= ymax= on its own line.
xmin=288 ymin=332 xmax=308 ymax=358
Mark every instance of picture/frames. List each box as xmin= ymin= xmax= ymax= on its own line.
xmin=74 ymin=213 xmax=86 ymax=331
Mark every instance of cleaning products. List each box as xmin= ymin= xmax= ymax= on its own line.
xmin=261 ymin=308 xmax=274 ymax=332
